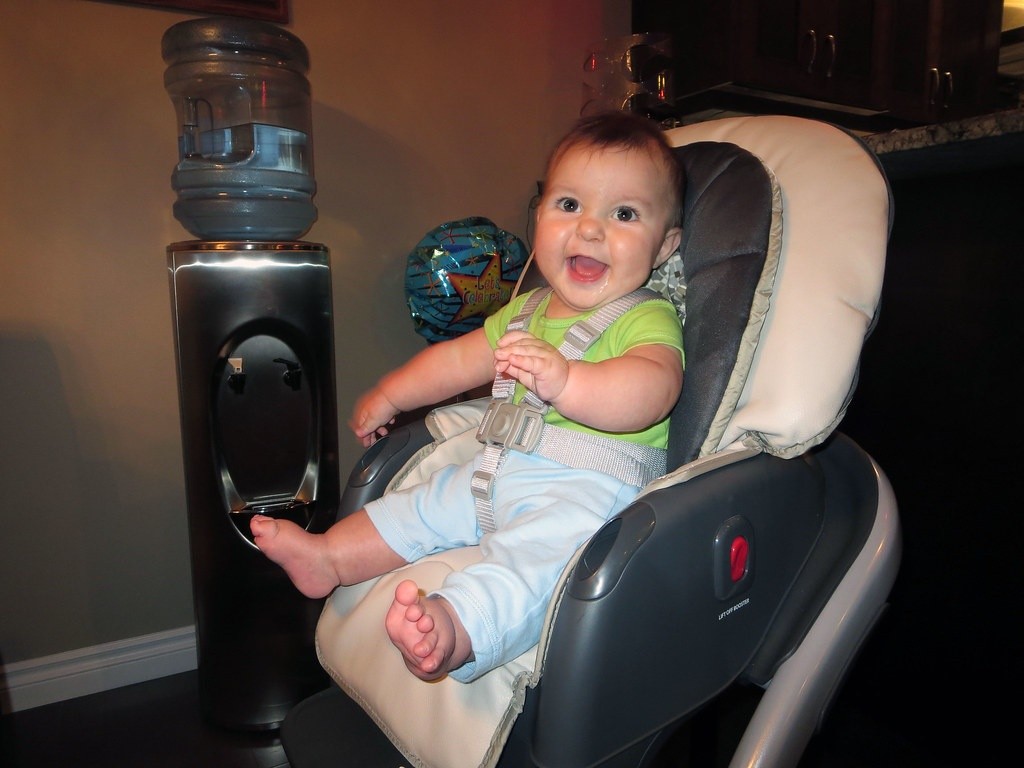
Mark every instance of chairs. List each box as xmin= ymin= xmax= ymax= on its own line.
xmin=279 ymin=117 xmax=904 ymax=767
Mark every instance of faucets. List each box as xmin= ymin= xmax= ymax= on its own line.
xmin=226 ymin=357 xmax=249 ymax=389
xmin=273 ymin=357 xmax=303 ymax=391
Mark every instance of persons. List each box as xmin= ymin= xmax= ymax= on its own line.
xmin=249 ymin=112 xmax=688 ymax=685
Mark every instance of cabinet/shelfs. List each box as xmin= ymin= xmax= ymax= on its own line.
xmin=633 ymin=0 xmax=1003 ymax=124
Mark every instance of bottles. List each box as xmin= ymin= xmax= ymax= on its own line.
xmin=160 ymin=17 xmax=317 ymax=240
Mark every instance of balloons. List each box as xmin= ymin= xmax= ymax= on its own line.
xmin=403 ymin=217 xmax=532 ymax=346
